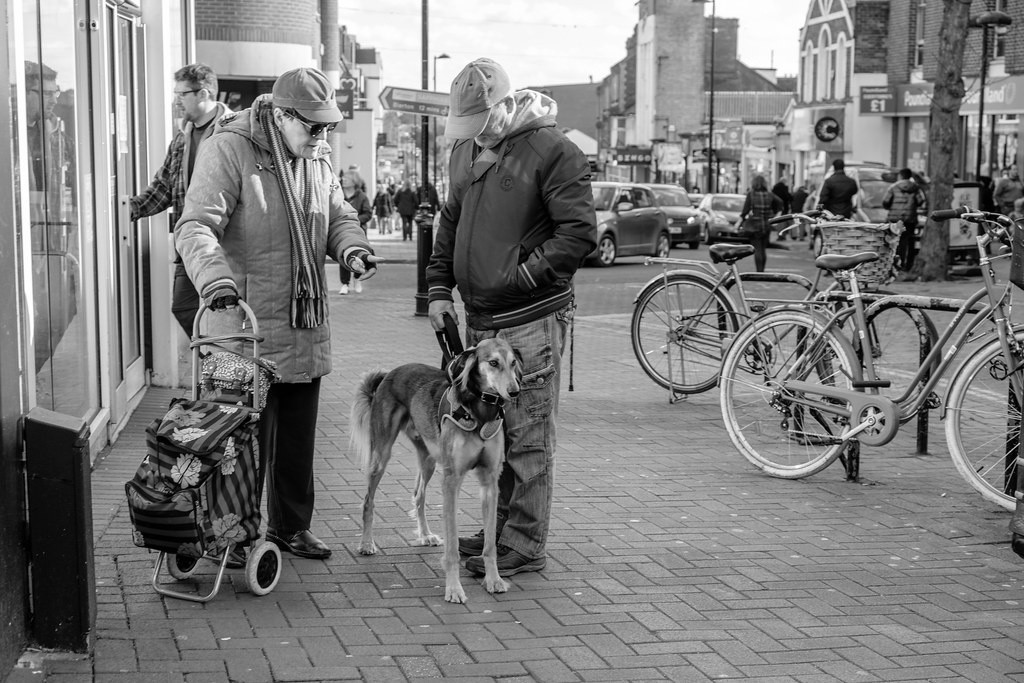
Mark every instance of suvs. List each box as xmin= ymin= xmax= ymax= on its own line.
xmin=805 ymin=164 xmax=928 ymax=272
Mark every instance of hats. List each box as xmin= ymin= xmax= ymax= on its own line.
xmin=272 ymin=68 xmax=343 ymax=122
xmin=444 ymin=59 xmax=511 ymax=138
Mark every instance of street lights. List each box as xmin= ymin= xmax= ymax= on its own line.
xmin=690 ymin=0 xmax=715 ymax=193
xmin=968 ymin=11 xmax=1013 ymax=182
xmin=434 ymin=54 xmax=450 ymax=187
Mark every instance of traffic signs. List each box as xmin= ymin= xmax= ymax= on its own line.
xmin=378 ymin=86 xmax=450 ymax=117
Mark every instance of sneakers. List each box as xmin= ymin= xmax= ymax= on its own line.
xmin=266 ymin=525 xmax=331 ymax=558
xmin=457 ymin=531 xmax=501 ymax=555
xmin=464 ymin=541 xmax=547 ymax=576
xmin=212 ymin=547 xmax=247 ymax=567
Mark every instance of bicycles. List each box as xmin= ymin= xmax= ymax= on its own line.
xmin=718 ymin=205 xmax=1024 ymax=514
xmin=631 ymin=210 xmax=944 ymax=426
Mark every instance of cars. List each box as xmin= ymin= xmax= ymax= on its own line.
xmin=587 ymin=182 xmax=749 ymax=269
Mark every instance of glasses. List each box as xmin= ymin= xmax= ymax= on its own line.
xmin=29 ymin=90 xmax=60 ymax=99
xmin=282 ymin=109 xmax=338 ymax=137
xmin=173 ymin=88 xmax=210 ymax=99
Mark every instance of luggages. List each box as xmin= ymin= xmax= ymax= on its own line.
xmin=126 ymin=299 xmax=281 ymax=602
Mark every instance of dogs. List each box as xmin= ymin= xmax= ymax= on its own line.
xmin=349 ymin=337 xmax=522 ymax=604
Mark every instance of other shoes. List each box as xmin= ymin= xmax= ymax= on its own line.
xmin=339 ymin=284 xmax=348 ymax=295
xmin=354 ymin=276 xmax=362 ymax=293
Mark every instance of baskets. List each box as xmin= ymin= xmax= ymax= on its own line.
xmin=1009 ymin=218 xmax=1024 ymax=291
xmin=820 ymin=222 xmax=902 ymax=284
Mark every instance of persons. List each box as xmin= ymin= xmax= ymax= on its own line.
xmin=699 ymin=156 xmax=1024 ymax=277
xmin=22 ymin=63 xmax=81 ymax=366
xmin=174 ymin=68 xmax=386 ymax=557
xmin=427 ymin=58 xmax=600 ymax=577
xmin=124 ymin=64 xmax=442 ymax=357
